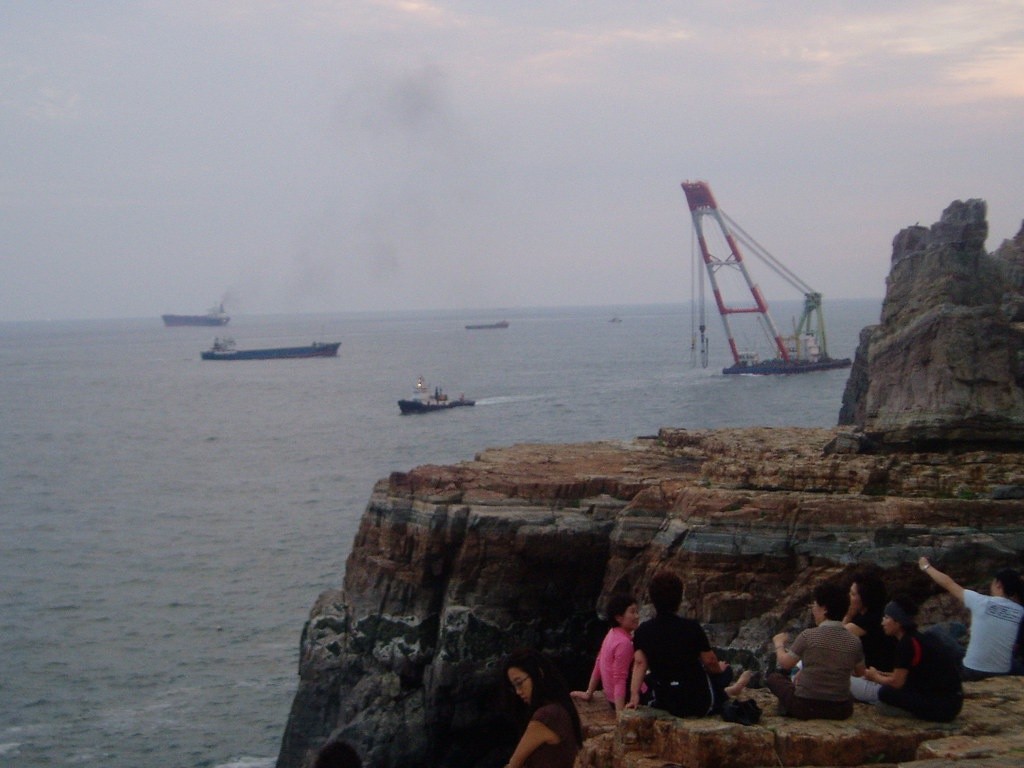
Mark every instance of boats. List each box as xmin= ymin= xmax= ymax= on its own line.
xmin=398 ymin=388 xmax=476 ymax=414
xmin=200 ymin=335 xmax=343 ymax=360
xmin=161 ymin=313 xmax=230 ymax=327
xmin=678 ymin=179 xmax=852 ymax=376
xmin=464 ymin=320 xmax=510 ymax=330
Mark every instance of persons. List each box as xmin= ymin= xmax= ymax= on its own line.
xmin=504 ymin=647 xmax=584 ymax=768
xmin=569 ymin=554 xmax=1022 ymax=723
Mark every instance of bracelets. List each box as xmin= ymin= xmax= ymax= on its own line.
xmin=774 ymin=645 xmax=785 ymax=650
xmin=586 ymin=689 xmax=593 ymax=696
xmin=880 ymin=671 xmax=887 ymax=676
xmin=845 ymin=612 xmax=856 ymax=621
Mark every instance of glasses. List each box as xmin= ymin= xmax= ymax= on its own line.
xmin=511 ymin=675 xmax=530 ymax=689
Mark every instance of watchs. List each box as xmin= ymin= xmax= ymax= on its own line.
xmin=922 ymin=563 xmax=931 ymax=571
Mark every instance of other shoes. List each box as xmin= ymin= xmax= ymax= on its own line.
xmin=720 ymin=699 xmax=762 ymax=725
